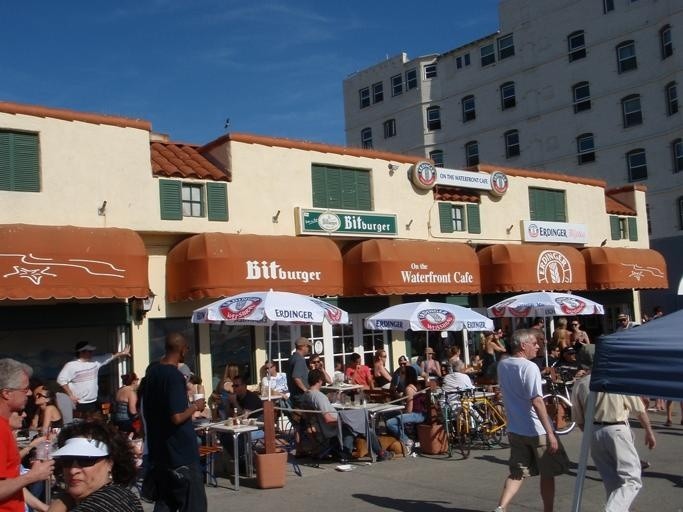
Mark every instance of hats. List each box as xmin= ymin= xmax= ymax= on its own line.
xmin=618 ymin=314 xmax=628 ymax=320
xmin=295 ymin=337 xmax=314 ymax=346
xmin=398 ymin=355 xmax=409 ymax=362
xmin=78 ymin=344 xmax=96 ymax=351
xmin=51 ymin=438 xmax=112 ymax=457
xmin=424 ymin=347 xmax=435 ymax=354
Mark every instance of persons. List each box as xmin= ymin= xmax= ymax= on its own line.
xmin=614 ymin=313 xmax=638 ymax=333
xmin=1 ymin=357 xmax=57 ymax=511
xmin=493 ymin=326 xmax=507 ymax=350
xmin=138 ymin=330 xmax=209 ymax=512
xmin=549 ymin=346 xmax=589 ymax=422
xmin=553 ymin=317 xmax=573 ymax=353
xmin=56 ymin=340 xmax=132 ymax=422
xmin=423 ymin=346 xmax=442 ymax=378
xmin=109 ymin=371 xmax=141 ymax=440
xmin=571 ymin=344 xmax=656 ymax=512
xmin=570 ymin=317 xmax=590 ymax=347
xmin=492 ymin=328 xmax=571 ymax=512
xmin=654 ymin=398 xmax=666 ymax=411
xmin=441 ymin=366 xmax=449 ymax=375
xmin=541 ymin=342 xmax=561 ymax=396
xmin=301 ymin=369 xmax=393 ymax=465
xmin=187 ymin=375 xmax=221 ymax=473
xmin=345 ymin=352 xmax=375 ymax=403
xmin=307 ymin=352 xmax=334 ymax=386
xmin=213 ymin=375 xmax=263 ymax=477
xmin=664 ymin=400 xmax=683 ymax=427
xmin=286 ymin=336 xmax=319 ymax=459
xmin=478 ymin=331 xmax=506 ymax=362
xmin=652 ymin=306 xmax=664 ymax=321
xmin=334 ymin=362 xmax=346 ymax=382
xmin=134 ymin=360 xmax=192 ymax=478
xmin=28 ymin=385 xmax=65 ymax=436
xmin=8 ymin=403 xmax=57 ymax=512
xmin=386 ymin=365 xmax=431 ymax=456
xmin=47 ymin=418 xmax=145 ymax=512
xmin=386 ymin=355 xmax=418 ymax=440
xmin=439 ymin=359 xmax=475 ymax=445
xmin=216 ymin=362 xmax=239 ymax=461
xmin=472 ymin=353 xmax=483 ymax=369
xmin=639 ymin=397 xmax=651 ymax=470
xmin=373 ymin=349 xmax=393 ymax=386
xmin=260 ymin=358 xmax=298 ymax=429
xmin=529 ymin=317 xmax=548 ymax=372
xmin=445 ymin=345 xmax=467 ymax=372
xmin=412 ymin=355 xmax=429 ymax=383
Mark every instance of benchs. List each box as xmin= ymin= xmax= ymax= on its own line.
xmin=199 ymin=444 xmax=224 ymax=486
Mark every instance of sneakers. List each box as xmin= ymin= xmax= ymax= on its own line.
xmin=492 ymin=505 xmax=504 ymax=512
xmin=404 ymin=439 xmax=414 ymax=457
xmin=377 ymin=451 xmax=395 ymax=462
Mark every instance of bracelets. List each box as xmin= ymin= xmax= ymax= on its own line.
xmin=64 ymin=390 xmax=73 ymax=397
xmin=123 ymin=351 xmax=126 ymax=357
xmin=193 ymin=403 xmax=199 ymax=412
xmin=24 ymin=447 xmax=30 ymax=456
xmin=495 ymin=338 xmax=500 ymax=344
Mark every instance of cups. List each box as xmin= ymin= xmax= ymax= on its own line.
xmin=36 ymin=441 xmax=53 ymax=461
xmin=241 ymin=418 xmax=250 ymax=425
xmin=193 ymin=393 xmax=205 ymax=404
xmin=251 ymin=418 xmax=258 ymax=426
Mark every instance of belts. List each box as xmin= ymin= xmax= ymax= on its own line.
xmin=593 ymin=421 xmax=625 ymax=426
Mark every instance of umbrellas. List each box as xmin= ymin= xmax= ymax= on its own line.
xmin=364 ymin=298 xmax=495 ymax=379
xmin=189 ymin=287 xmax=348 ymax=400
xmin=486 ymin=289 xmax=606 ymax=368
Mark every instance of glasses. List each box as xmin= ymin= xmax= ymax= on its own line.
xmin=382 ymin=355 xmax=386 ymax=357
xmin=265 ymin=364 xmax=275 ymax=370
xmin=8 ymin=384 xmax=28 ymax=393
xmin=572 ymin=324 xmax=577 ymax=326
xmin=311 ymin=361 xmax=320 ymax=364
xmin=11 ymin=409 xmax=25 ymax=416
xmin=232 ymin=384 xmax=244 ymax=387
xmin=59 ymin=456 xmax=108 ymax=468
xmin=35 ymin=393 xmax=50 ymax=399
xmin=399 ymin=363 xmax=406 ymax=366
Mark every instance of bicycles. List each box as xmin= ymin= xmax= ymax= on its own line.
xmin=441 ymin=360 xmax=578 ymax=457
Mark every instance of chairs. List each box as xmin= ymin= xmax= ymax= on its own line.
xmin=279 ymin=396 xmax=375 ymax=468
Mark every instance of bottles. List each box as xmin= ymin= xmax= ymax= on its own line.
xmin=233 ymin=408 xmax=238 ymax=426
